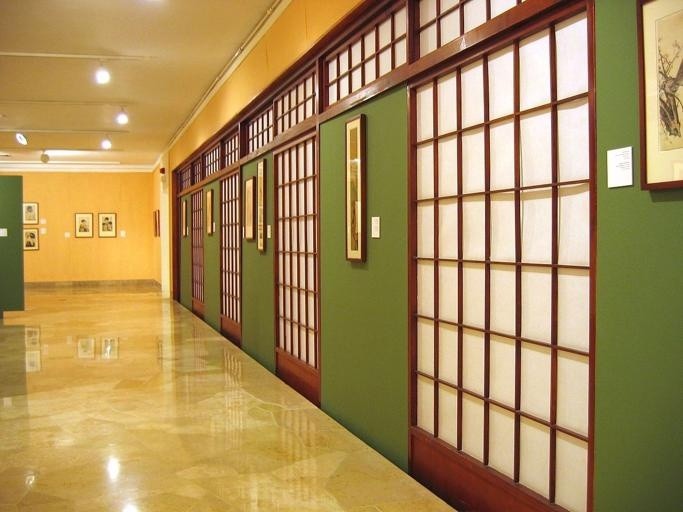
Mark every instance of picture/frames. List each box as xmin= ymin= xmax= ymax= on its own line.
xmin=73 ymin=332 xmax=121 ymax=362
xmin=24 ymin=324 xmax=42 ymax=374
xmin=633 ymin=0 xmax=682 ymax=193
xmin=74 ymin=212 xmax=94 ymax=238
xmin=243 ymin=175 xmax=256 ymax=241
xmin=343 ymin=112 xmax=367 ymax=264
xmin=205 ymin=188 xmax=213 ymax=236
xmin=152 ymin=208 xmax=160 ymax=239
xmin=181 ymin=200 xmax=187 ymax=237
xmin=97 ymin=213 xmax=117 ymax=238
xmin=22 ymin=227 xmax=39 ymax=251
xmin=22 ymin=201 xmax=39 ymax=225
xmin=255 ymin=157 xmax=266 ymax=254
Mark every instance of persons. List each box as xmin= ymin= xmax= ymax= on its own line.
xmin=78 ymin=218 xmax=89 ymax=233
xmin=24 ymin=205 xmax=36 ymax=221
xmin=101 ymin=216 xmax=113 ymax=232
xmin=25 ymin=233 xmax=36 ymax=247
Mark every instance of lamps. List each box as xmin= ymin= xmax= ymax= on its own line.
xmin=101 ymin=131 xmax=112 ymax=150
xmin=115 ymin=106 xmax=130 ymax=127
xmin=39 ymin=149 xmax=50 ymax=165
xmin=95 ymin=55 xmax=109 ymax=85
